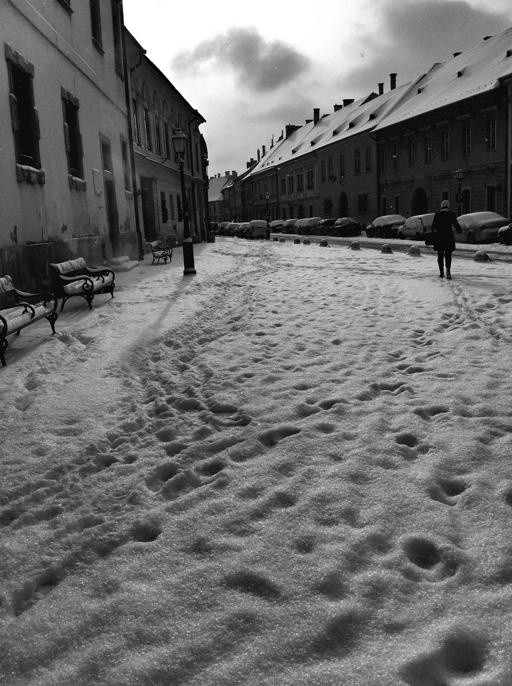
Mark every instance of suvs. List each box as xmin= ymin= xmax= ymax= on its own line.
xmin=296 ymin=216 xmax=321 ymax=234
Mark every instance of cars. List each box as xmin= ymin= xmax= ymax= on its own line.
xmin=366 ymin=214 xmax=405 ymax=238
xmin=496 ymin=223 xmax=512 ymax=244
xmin=328 ymin=216 xmax=362 ymax=236
xmin=283 ymin=219 xmax=298 ymax=234
xmin=395 ymin=211 xmax=436 ymax=240
xmin=270 ymin=219 xmax=283 ymax=233
xmin=318 ymin=219 xmax=334 ymax=235
xmin=451 ymin=210 xmax=509 ymax=243
xmin=208 ymin=218 xmax=270 ymax=240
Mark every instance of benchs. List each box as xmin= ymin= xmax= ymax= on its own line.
xmin=45 ymin=255 xmax=119 ymax=315
xmin=147 ymin=240 xmax=175 ymax=266
xmin=1 ymin=269 xmax=62 ymax=368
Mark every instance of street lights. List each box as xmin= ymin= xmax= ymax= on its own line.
xmin=454 ymin=168 xmax=464 ymax=216
xmin=264 ymin=191 xmax=270 ymax=218
xmin=170 ymin=127 xmax=196 ymax=275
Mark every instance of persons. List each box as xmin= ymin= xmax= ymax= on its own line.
xmin=430 ymin=199 xmax=462 ymax=279
